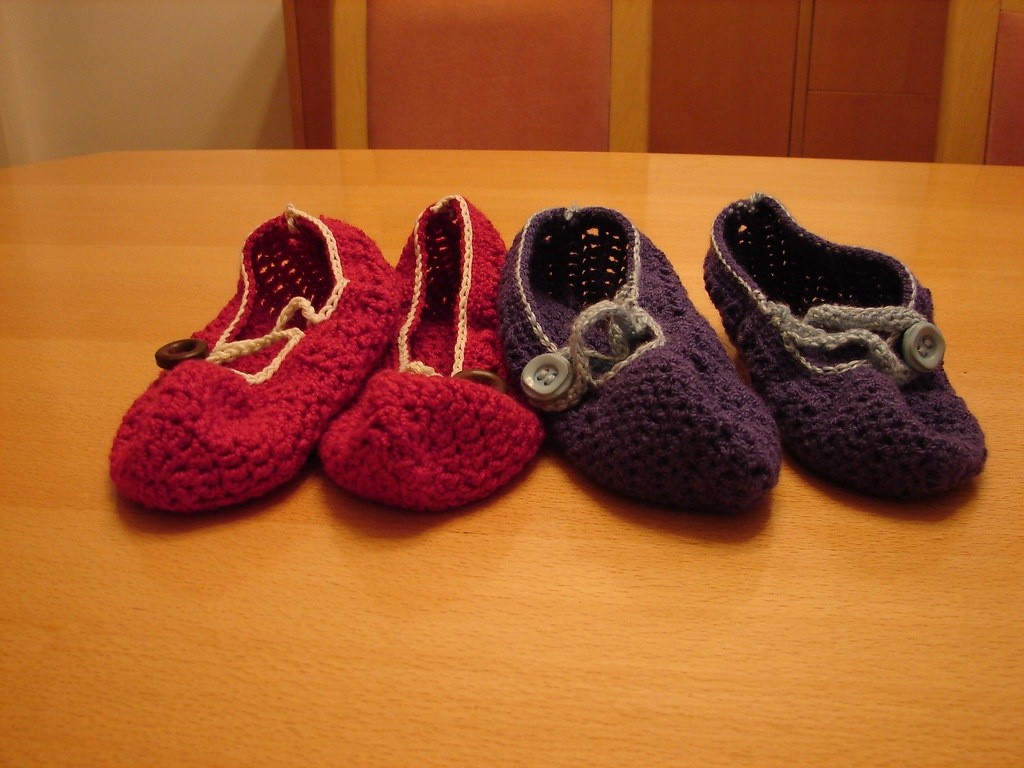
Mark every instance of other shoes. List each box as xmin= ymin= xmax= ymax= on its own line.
xmin=498 ymin=206 xmax=781 ymax=514
xmin=109 ymin=203 xmax=401 ymax=514
xmin=317 ymin=196 xmax=544 ymax=514
xmin=704 ymin=195 xmax=989 ymax=497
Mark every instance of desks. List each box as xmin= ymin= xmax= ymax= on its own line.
xmin=0 ymin=148 xmax=1024 ymax=768
